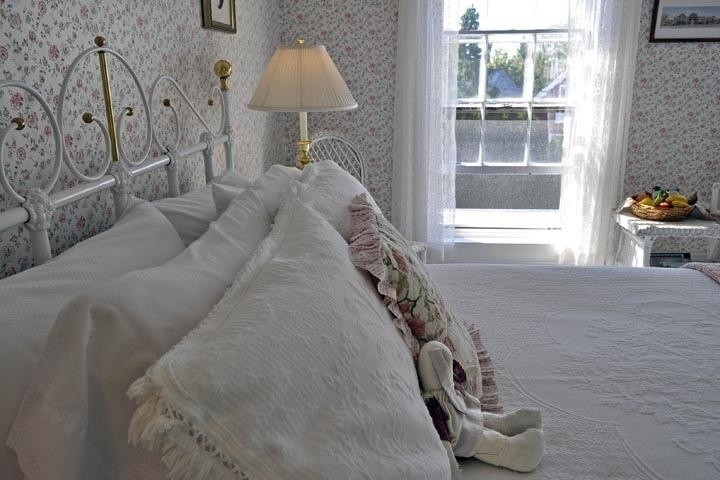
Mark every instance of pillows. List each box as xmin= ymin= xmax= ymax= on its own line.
xmin=0 ymin=196 xmax=189 ymax=480
xmin=7 ymin=187 xmax=273 ymax=480
xmin=287 ymin=160 xmax=383 ymax=236
xmin=349 ymin=191 xmax=503 ymax=412
xmin=152 ymin=164 xmax=302 ymax=245
xmin=124 ymin=200 xmax=464 ymax=480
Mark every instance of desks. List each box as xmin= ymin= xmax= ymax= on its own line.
xmin=614 ymin=213 xmax=720 ymax=267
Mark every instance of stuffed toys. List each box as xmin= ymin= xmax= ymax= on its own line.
xmin=414 ymin=339 xmax=544 ymax=473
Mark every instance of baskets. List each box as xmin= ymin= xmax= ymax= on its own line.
xmin=629 ymin=198 xmax=697 ymax=221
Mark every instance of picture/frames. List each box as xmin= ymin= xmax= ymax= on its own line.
xmin=201 ymin=0 xmax=236 ymax=33
xmin=649 ymin=0 xmax=720 ymax=42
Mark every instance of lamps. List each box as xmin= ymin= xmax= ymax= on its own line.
xmin=247 ymin=39 xmax=358 ymax=169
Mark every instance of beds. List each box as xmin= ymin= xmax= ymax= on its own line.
xmin=0 ymin=36 xmax=720 ymax=480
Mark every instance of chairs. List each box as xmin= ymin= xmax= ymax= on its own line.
xmin=309 ymin=130 xmax=427 ymax=263
